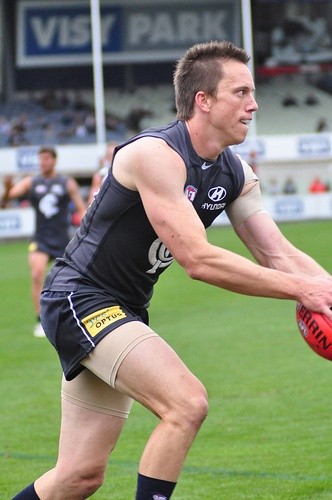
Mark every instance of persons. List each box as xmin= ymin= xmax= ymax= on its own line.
xmin=1 ymin=82 xmax=154 ymax=146
xmin=267 ymin=172 xmax=329 ymax=195
xmin=280 ymin=88 xmax=330 ymax=132
xmin=9 ymin=144 xmax=89 ymax=338
xmin=88 ymin=140 xmax=119 ymax=207
xmin=8 ymin=40 xmax=332 ymax=500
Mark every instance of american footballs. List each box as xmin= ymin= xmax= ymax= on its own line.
xmin=296 ymin=301 xmax=332 ymax=362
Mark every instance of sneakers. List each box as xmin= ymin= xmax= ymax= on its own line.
xmin=34 ymin=322 xmax=46 ymax=338
